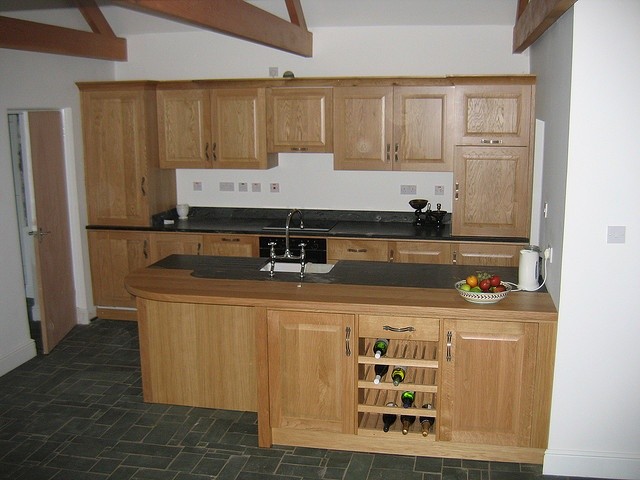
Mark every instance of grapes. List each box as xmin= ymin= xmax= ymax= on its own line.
xmin=471 ymin=270 xmax=495 ymax=285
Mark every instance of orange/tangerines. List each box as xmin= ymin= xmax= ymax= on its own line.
xmin=466 ymin=274 xmax=478 ymax=287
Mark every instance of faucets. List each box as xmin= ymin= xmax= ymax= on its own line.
xmin=266 ymin=208 xmax=306 ymax=278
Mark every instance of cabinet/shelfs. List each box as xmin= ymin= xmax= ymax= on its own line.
xmin=152 ymin=230 xmax=259 ymax=266
xmin=326 ymin=237 xmax=451 ymax=266
xmin=453 ymin=244 xmax=532 ymax=267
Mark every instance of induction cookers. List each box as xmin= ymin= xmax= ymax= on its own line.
xmin=261 ymin=218 xmax=338 ymax=232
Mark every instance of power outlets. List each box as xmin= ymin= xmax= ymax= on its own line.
xmin=547 ymin=245 xmax=553 ymax=263
xmin=400 ymin=184 xmax=418 ymax=196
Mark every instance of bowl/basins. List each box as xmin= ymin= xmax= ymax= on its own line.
xmin=455 ymin=279 xmax=512 ymax=304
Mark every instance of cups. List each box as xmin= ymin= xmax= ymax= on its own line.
xmin=176 ymin=203 xmax=189 ymax=220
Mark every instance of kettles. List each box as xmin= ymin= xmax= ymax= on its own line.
xmin=518 ymin=249 xmax=540 ymax=292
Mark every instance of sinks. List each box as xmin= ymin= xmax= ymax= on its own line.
xmin=259 ymin=260 xmax=335 ymax=274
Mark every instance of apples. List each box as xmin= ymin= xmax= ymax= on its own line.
xmin=470 ymin=286 xmax=482 ymax=292
xmin=479 ymin=279 xmax=490 ymax=290
xmin=482 ymin=289 xmax=492 ymax=293
xmin=489 ymin=276 xmax=500 ymax=287
xmin=493 ymin=285 xmax=508 ymax=293
xmin=459 ymin=284 xmax=470 ymax=292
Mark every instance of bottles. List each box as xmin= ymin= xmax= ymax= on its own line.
xmin=391 ymin=366 xmax=407 ymax=387
xmin=401 ymin=405 xmax=416 ymax=435
xmin=383 ymin=402 xmax=397 ymax=433
xmin=374 ymin=366 xmax=390 ymax=384
xmin=374 ymin=339 xmax=390 ymax=359
xmin=420 ymin=404 xmax=435 ymax=437
xmin=401 ymin=391 xmax=415 ymax=409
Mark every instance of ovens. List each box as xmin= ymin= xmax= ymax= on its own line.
xmin=259 ymin=238 xmax=326 ymax=264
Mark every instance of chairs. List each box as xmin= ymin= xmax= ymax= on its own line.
xmin=265 ymin=77 xmax=333 ymax=152
xmin=454 ymin=74 xmax=536 ymax=146
xmin=154 ymin=78 xmax=279 ymax=171
xmin=87 ymin=228 xmax=147 ymax=319
xmin=451 ymin=143 xmax=530 ymax=243
xmin=440 ymin=318 xmax=553 ymax=464
xmin=258 ymin=302 xmax=439 ymax=460
xmin=331 ymin=75 xmax=454 ymax=171
xmin=75 ymin=80 xmax=177 ymax=225
